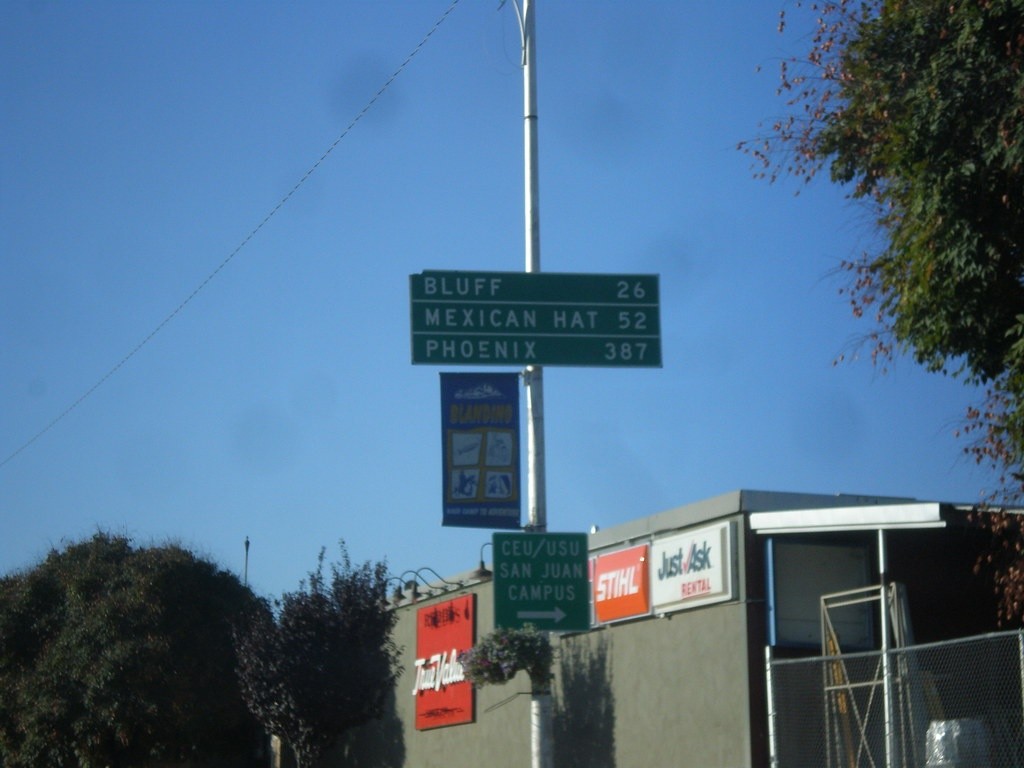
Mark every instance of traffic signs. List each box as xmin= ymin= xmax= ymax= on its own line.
xmin=491 ymin=530 xmax=593 ymax=634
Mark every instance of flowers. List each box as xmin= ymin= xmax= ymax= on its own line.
xmin=454 ymin=620 xmax=549 ymax=689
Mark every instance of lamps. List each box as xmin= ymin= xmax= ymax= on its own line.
xmin=468 ymin=542 xmax=494 ymax=580
xmin=375 ymin=567 xmax=465 ymax=607
